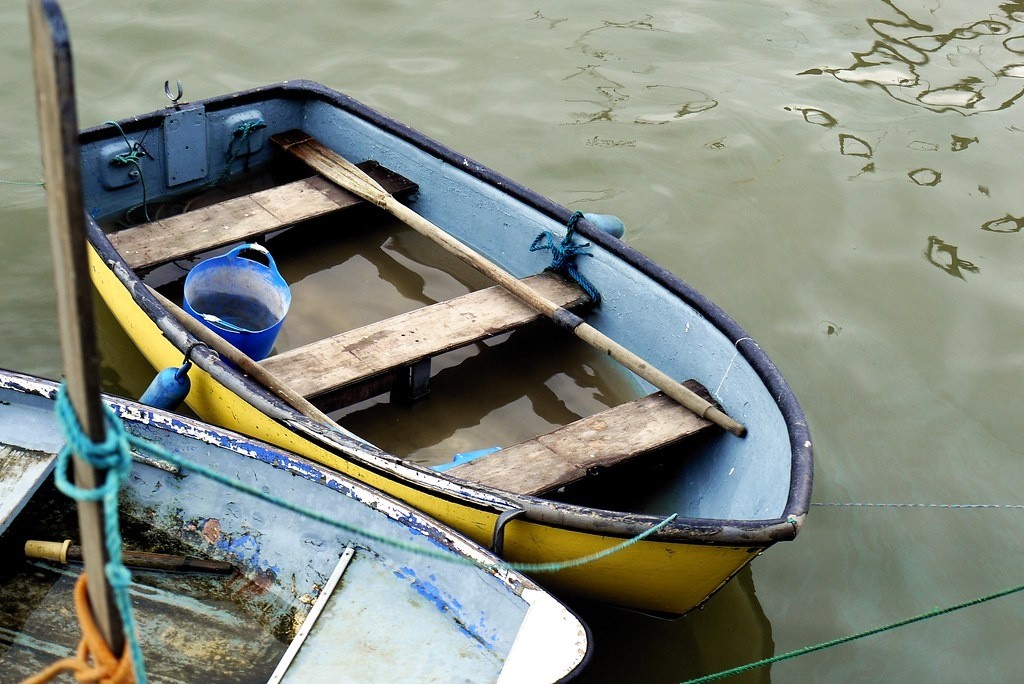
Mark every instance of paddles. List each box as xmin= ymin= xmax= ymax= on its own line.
xmin=142 ymin=280 xmax=386 ymax=450
xmin=271 ymin=126 xmax=750 ymax=441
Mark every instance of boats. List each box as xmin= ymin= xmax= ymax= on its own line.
xmin=75 ymin=80 xmax=814 ymax=620
xmin=0 ymin=367 xmax=594 ymax=684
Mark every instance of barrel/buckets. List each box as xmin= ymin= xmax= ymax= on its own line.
xmin=183 ymin=243 xmax=292 ymax=362
